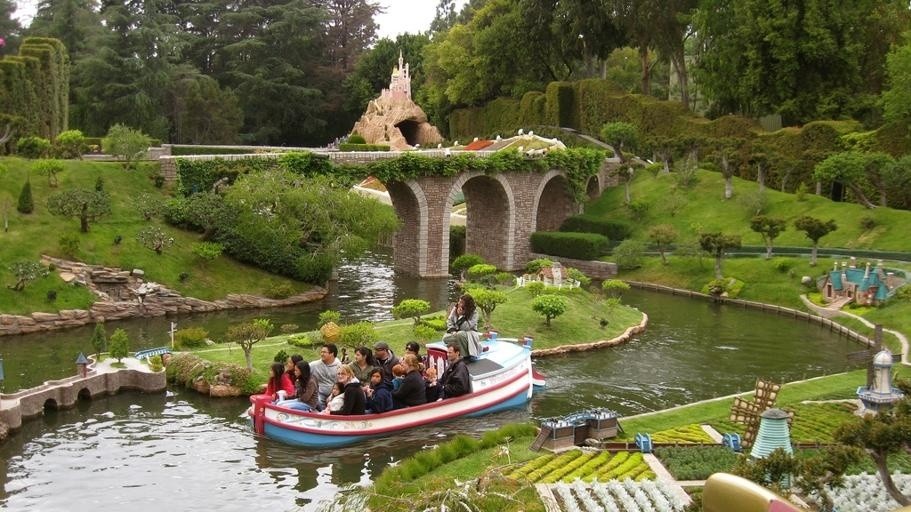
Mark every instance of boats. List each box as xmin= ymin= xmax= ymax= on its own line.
xmin=247 ymin=331 xmax=547 ymax=451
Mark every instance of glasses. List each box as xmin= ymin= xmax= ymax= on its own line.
xmin=406 ymin=346 xmax=414 ymax=351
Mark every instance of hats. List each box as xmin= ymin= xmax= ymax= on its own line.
xmin=373 ymin=342 xmax=390 ymax=350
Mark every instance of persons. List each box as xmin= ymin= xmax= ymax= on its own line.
xmin=443 ymin=293 xmax=489 ymax=361
xmin=263 ymin=338 xmax=469 ymax=416
